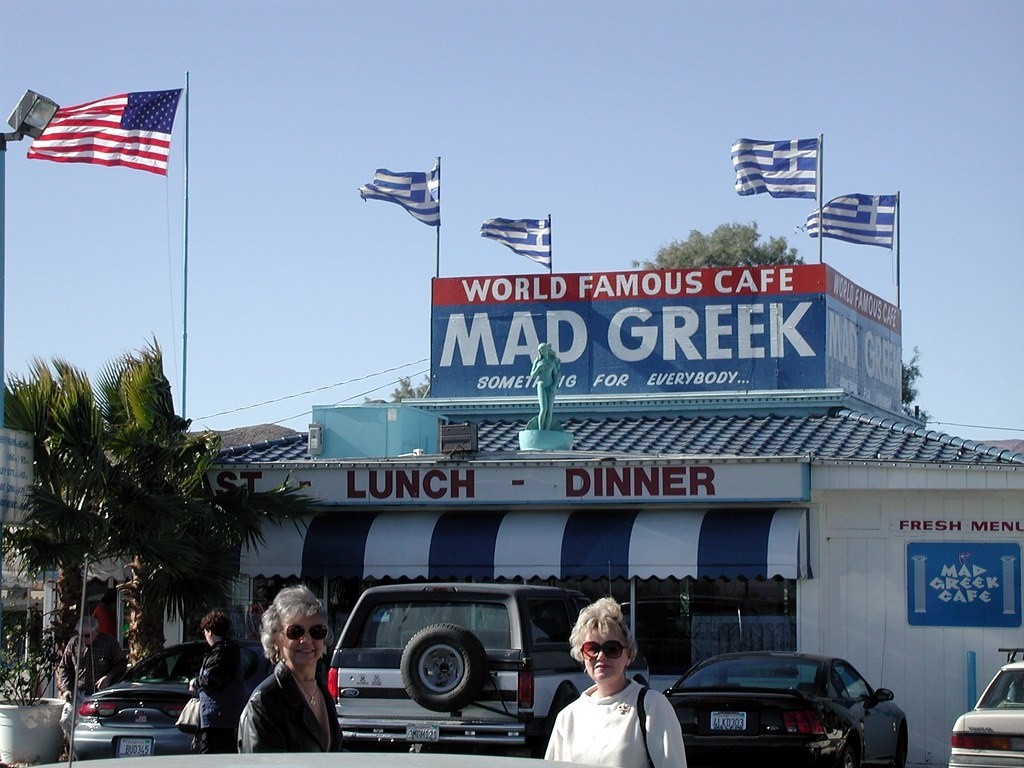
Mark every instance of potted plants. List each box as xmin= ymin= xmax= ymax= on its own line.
xmin=0 ymin=599 xmax=79 ymax=766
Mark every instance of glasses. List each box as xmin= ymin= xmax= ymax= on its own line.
xmin=81 ymin=630 xmax=95 ymax=639
xmin=278 ymin=624 xmax=327 ymax=641
xmin=579 ymin=639 xmax=628 ymax=660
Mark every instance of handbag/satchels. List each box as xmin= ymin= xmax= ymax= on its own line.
xmin=175 ymin=689 xmax=201 ymax=733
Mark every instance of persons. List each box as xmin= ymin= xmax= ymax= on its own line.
xmin=237 ymin=585 xmax=343 ymax=752
xmin=189 ymin=611 xmax=241 ymax=752
xmin=530 ymin=342 xmax=559 ymax=430
xmin=543 ymin=599 xmax=688 ymax=768
xmin=57 ymin=615 xmax=126 ymax=739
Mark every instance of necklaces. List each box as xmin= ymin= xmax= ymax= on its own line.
xmin=302 ymin=687 xmax=319 ymax=705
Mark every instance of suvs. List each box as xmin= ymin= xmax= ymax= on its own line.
xmin=326 ymin=581 xmax=651 ymax=760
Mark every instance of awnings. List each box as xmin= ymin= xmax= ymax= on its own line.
xmin=229 ymin=508 xmax=813 ymax=581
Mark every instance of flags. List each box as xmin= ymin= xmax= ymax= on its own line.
xmin=730 ymin=138 xmax=818 ymax=199
xmin=358 ymin=168 xmax=440 ymax=226
xmin=479 ymin=218 xmax=550 ymax=269
xmin=806 ymin=194 xmax=896 ymax=249
xmin=27 ymin=89 xmax=183 ymax=176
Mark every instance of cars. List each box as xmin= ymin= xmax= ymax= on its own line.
xmin=662 ymin=650 xmax=908 ymax=768
xmin=72 ymin=637 xmax=330 ymax=762
xmin=947 ymin=648 xmax=1024 ymax=768
